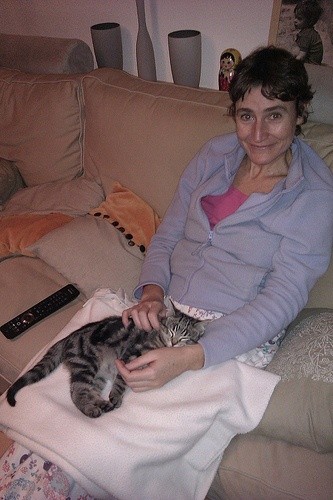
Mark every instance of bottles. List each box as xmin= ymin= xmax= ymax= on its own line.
xmin=135 ymin=0 xmax=157 ymax=82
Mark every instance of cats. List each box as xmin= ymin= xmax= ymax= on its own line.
xmin=7 ymin=296 xmax=217 ymax=419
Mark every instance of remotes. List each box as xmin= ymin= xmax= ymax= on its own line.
xmin=0 ymin=284 xmax=80 ymax=339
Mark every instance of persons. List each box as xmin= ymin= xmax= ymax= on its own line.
xmin=294 ymin=0 xmax=323 ymax=64
xmin=218 ymin=48 xmax=241 ymax=91
xmin=114 ymin=45 xmax=333 ymax=392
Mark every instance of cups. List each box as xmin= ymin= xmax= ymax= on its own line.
xmin=91 ymin=23 xmax=123 ymax=70
xmin=167 ymin=30 xmax=202 ymax=87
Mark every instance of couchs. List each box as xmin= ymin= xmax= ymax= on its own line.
xmin=0 ymin=68 xmax=332 ymax=500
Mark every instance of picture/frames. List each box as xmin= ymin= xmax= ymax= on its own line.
xmin=268 ymin=0 xmax=333 ymax=68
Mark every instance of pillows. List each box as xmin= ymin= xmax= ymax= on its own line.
xmin=0 ymin=182 xmax=105 ymax=257
xmin=28 ymin=181 xmax=160 ymax=298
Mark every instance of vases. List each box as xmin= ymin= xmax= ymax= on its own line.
xmin=91 ymin=22 xmax=123 ymax=71
xmin=169 ymin=29 xmax=201 ymax=90
